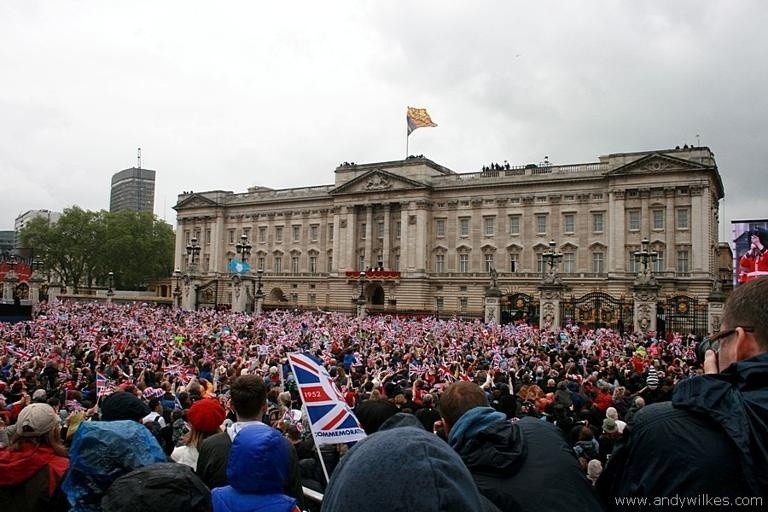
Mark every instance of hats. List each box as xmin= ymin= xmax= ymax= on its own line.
xmin=597 ymin=345 xmax=659 ymax=434
xmin=144 ymin=387 xmax=166 ymax=397
xmin=270 ymin=366 xmax=278 ymax=374
xmin=188 ymin=397 xmax=225 ymax=430
xmin=0 ymin=389 xmax=62 ymax=436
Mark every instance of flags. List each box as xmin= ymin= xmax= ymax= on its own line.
xmin=408 ymin=108 xmax=438 ymax=137
xmin=229 ymin=259 xmax=250 ymax=274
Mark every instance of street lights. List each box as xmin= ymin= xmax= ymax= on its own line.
xmin=32 ymin=254 xmax=45 ymax=270
xmin=632 ymin=235 xmax=659 ymax=276
xmin=356 ymin=270 xmax=368 ymax=301
xmin=4 ymin=254 xmax=19 ymax=270
xmin=105 ymin=268 xmax=117 ymax=296
xmin=173 ymin=267 xmax=182 ymax=293
xmin=539 ymin=239 xmax=564 ymax=273
xmin=253 ymin=268 xmax=265 ymax=298
xmin=184 ymin=236 xmax=202 ymax=265
xmin=235 ymin=231 xmax=253 ymax=263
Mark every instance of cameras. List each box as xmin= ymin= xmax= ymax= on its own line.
xmin=696 ymin=338 xmax=720 ymax=367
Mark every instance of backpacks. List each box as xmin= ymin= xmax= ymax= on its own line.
xmin=578 ymin=440 xmax=599 ymax=464
xmin=144 ymin=415 xmax=174 ymax=451
xmin=299 ymin=458 xmax=315 ymax=478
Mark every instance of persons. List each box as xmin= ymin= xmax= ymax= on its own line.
xmin=738 ymin=227 xmax=768 ymax=282
xmin=0 ymin=276 xmax=768 ymax=512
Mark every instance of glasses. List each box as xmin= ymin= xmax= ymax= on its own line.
xmin=699 ymin=326 xmax=754 ymax=356
